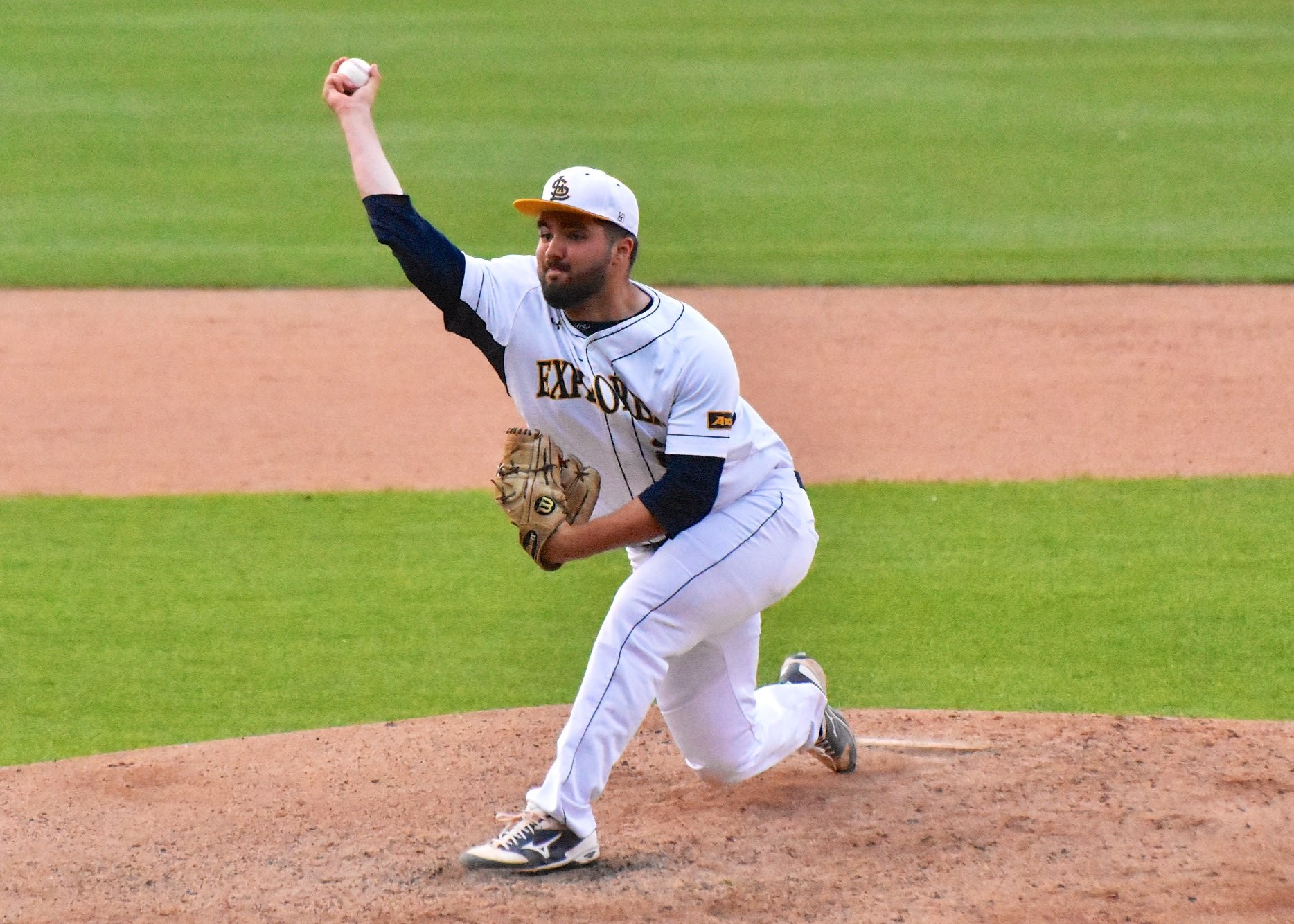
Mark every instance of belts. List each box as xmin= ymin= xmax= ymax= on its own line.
xmin=793 ymin=470 xmax=806 ymax=491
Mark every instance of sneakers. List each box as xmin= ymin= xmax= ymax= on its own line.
xmin=459 ymin=810 xmax=601 ymax=873
xmin=779 ymin=653 xmax=858 ymax=775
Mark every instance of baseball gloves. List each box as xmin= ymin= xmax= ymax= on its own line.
xmin=489 ymin=426 xmax=603 ymax=575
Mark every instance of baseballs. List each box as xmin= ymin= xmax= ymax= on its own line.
xmin=337 ymin=57 xmax=371 ymax=93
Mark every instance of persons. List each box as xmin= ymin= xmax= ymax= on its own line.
xmin=323 ymin=56 xmax=854 ymax=875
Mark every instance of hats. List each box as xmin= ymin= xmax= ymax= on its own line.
xmin=512 ymin=165 xmax=639 ymax=237
